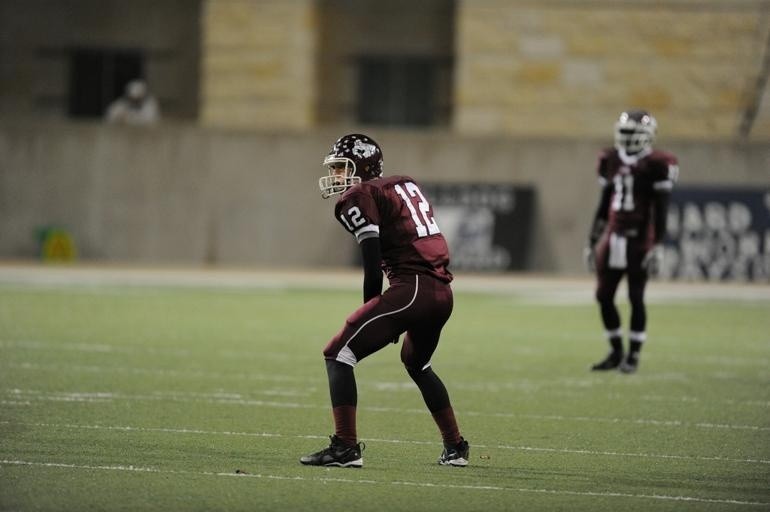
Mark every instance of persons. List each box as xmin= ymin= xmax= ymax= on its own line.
xmin=301 ymin=133 xmax=470 ymax=469
xmin=104 ymin=77 xmax=162 ymax=127
xmin=584 ymin=113 xmax=681 ymax=372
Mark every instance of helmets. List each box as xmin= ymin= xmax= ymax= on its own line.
xmin=318 ymin=134 xmax=384 ymax=200
xmin=614 ymin=110 xmax=655 ymax=154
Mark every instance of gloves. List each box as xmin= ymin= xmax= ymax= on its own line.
xmin=641 ymin=243 xmax=665 ymax=278
xmin=584 ymin=247 xmax=597 ymax=273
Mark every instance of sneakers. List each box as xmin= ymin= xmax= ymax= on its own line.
xmin=438 ymin=441 xmax=469 ymax=467
xmin=592 ymin=355 xmax=638 ymax=372
xmin=300 ymin=435 xmax=364 ymax=468
